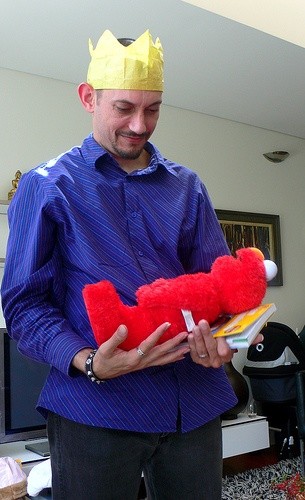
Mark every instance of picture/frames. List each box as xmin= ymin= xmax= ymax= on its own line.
xmin=212 ymin=209 xmax=283 ymax=287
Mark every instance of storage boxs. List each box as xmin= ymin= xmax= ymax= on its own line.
xmin=0 ymin=456 xmax=27 ymax=500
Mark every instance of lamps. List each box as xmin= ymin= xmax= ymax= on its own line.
xmin=263 ymin=151 xmax=289 ymax=163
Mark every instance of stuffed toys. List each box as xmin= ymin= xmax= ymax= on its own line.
xmin=81 ymin=247 xmax=278 ymax=353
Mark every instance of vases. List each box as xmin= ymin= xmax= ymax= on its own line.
xmin=220 ymin=348 xmax=249 ymax=420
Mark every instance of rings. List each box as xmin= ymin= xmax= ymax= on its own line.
xmin=198 ymin=353 xmax=208 ymax=358
xmin=136 ymin=347 xmax=147 ymax=357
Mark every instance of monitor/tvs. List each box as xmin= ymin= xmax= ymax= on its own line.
xmin=0 ymin=327 xmax=50 ymax=456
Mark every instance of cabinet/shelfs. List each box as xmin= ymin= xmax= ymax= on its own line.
xmin=0 ymin=200 xmax=11 ymax=269
xmin=26 ymin=413 xmax=271 ymax=477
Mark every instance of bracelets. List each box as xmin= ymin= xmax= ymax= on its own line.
xmin=84 ymin=348 xmax=109 ymax=385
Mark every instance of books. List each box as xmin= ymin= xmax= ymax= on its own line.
xmin=209 ymin=303 xmax=277 ymax=349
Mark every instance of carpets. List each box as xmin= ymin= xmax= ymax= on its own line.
xmin=221 ymin=456 xmax=305 ymax=500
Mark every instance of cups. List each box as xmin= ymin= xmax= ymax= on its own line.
xmin=245 ymin=403 xmax=257 ymax=418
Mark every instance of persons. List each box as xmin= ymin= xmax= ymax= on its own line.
xmin=0 ymin=29 xmax=266 ymax=500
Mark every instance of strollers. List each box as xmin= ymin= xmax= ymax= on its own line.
xmin=242 ymin=321 xmax=305 ymax=460
